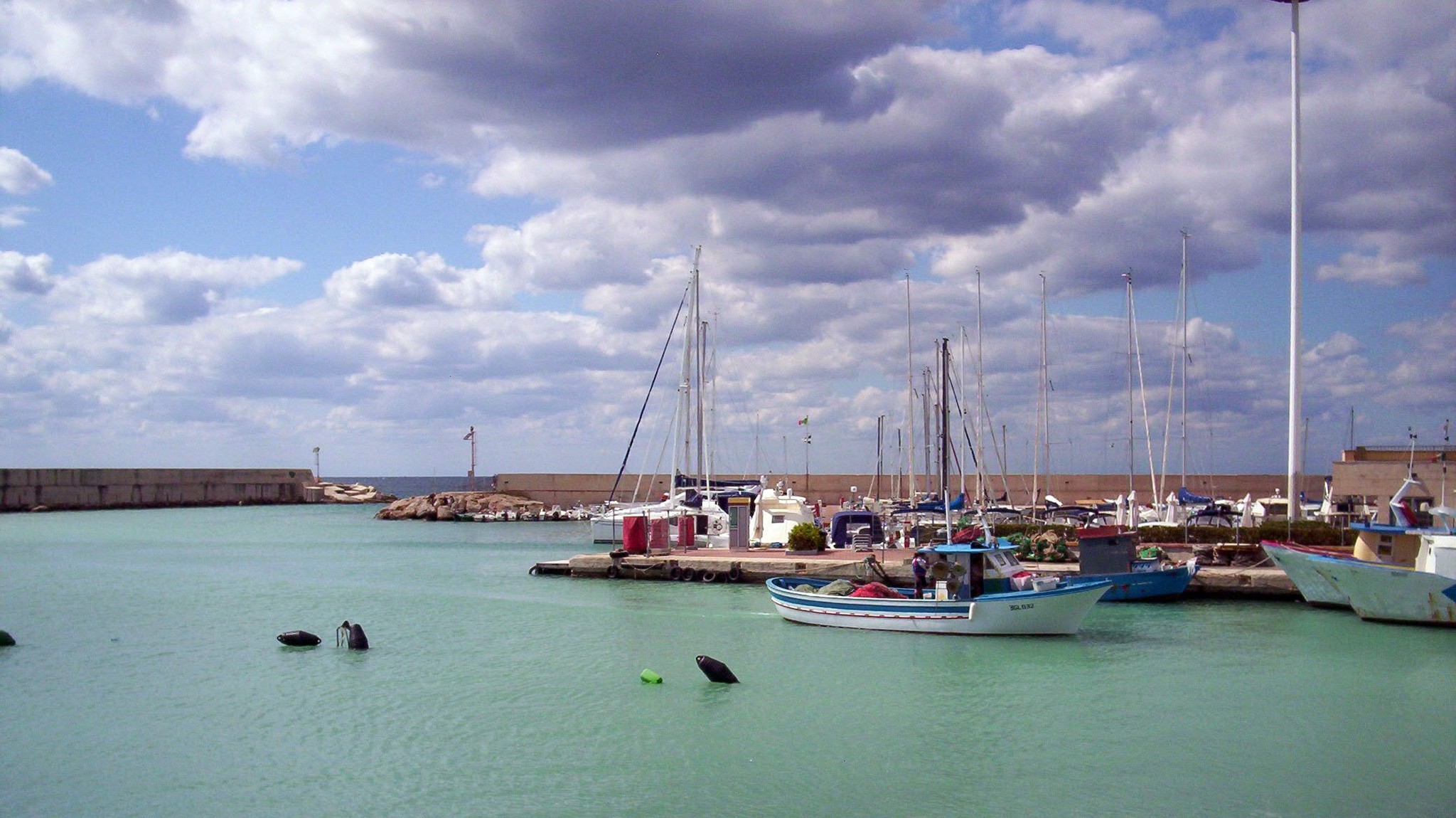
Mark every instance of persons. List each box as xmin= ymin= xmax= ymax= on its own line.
xmin=912 ymin=552 xmax=928 ymax=600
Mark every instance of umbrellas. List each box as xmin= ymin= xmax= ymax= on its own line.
xmin=1127 ymin=491 xmax=1139 ymax=531
xmin=1165 ymin=491 xmax=1180 ymax=523
xmin=752 ymin=494 xmax=764 ymax=542
xmin=1238 ymin=492 xmax=1254 ymax=528
xmin=1114 ymin=495 xmax=1126 ymax=534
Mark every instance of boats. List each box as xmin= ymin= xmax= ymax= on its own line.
xmin=1255 ymin=426 xmax=1431 ymax=607
xmin=765 ymin=484 xmax=1114 ymax=639
xmin=1303 ymin=450 xmax=1456 ymax=624
xmin=977 ymin=516 xmax=1206 ymax=602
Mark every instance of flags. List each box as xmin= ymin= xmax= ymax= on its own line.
xmin=802 ymin=435 xmax=811 ymax=444
xmin=798 ymin=417 xmax=809 ymax=426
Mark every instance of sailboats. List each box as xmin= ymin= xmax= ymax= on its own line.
xmin=590 ymin=240 xmax=1236 ymax=543
